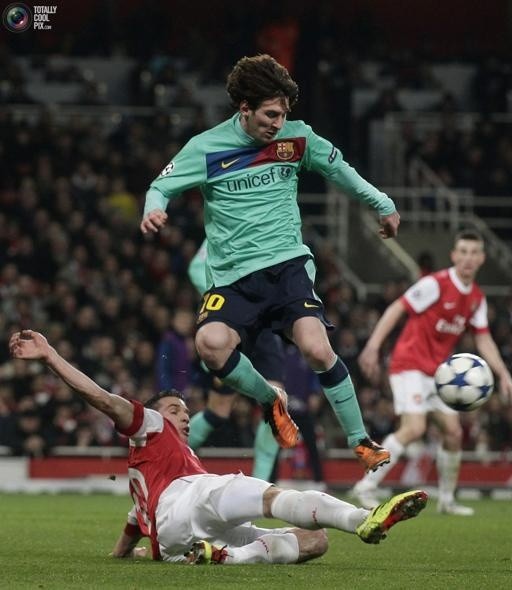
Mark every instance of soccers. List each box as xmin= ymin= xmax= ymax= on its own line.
xmin=435 ymin=353 xmax=494 ymax=412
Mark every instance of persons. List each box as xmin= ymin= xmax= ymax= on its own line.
xmin=1 ymin=49 xmax=511 ymax=516
xmin=9 ymin=328 xmax=427 ymax=565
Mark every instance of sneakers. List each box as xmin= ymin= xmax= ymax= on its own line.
xmin=353 ymin=479 xmax=380 ymax=509
xmin=436 ymin=496 xmax=474 ymax=518
xmin=354 ymin=436 xmax=391 ymax=474
xmin=356 ymin=489 xmax=428 ymax=544
xmin=184 ymin=539 xmax=233 ymax=565
xmin=258 ymin=385 xmax=301 ymax=449
